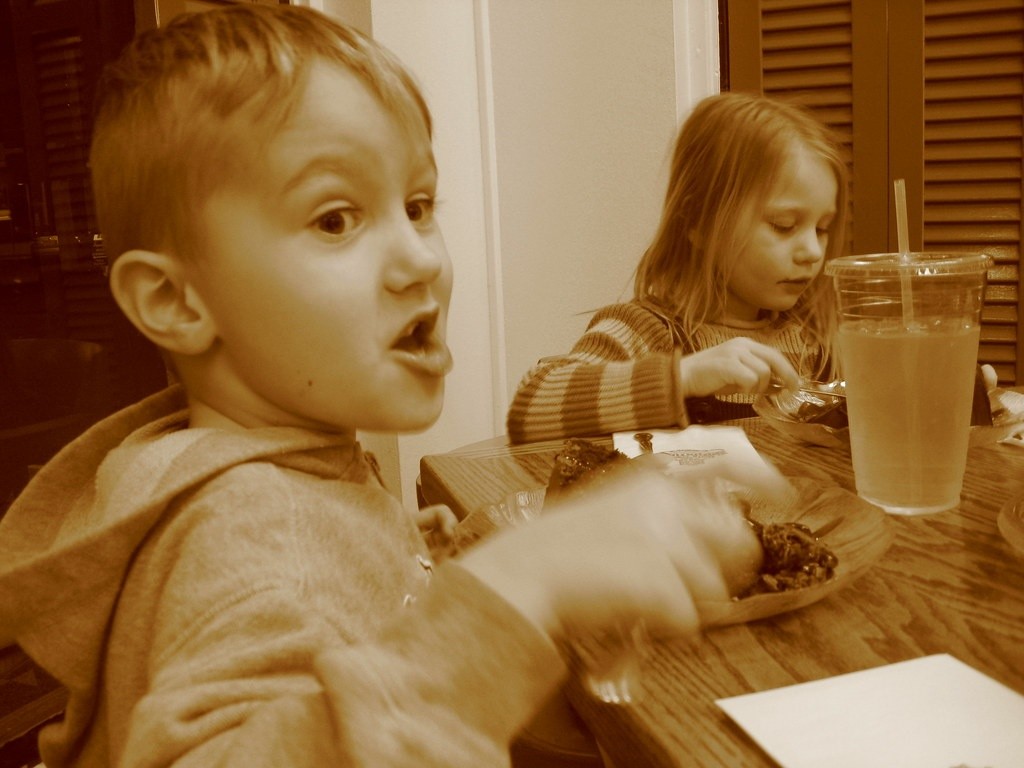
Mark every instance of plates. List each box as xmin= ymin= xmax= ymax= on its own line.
xmin=713 ymin=652 xmax=1024 ymax=768
xmin=753 ymin=378 xmax=1024 ymax=450
xmin=436 ymin=448 xmax=893 ymax=624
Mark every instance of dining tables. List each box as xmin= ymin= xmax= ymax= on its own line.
xmin=416 ymin=385 xmax=1024 ymax=767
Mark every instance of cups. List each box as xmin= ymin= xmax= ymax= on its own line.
xmin=825 ymin=251 xmax=991 ymax=514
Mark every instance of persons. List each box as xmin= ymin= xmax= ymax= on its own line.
xmin=0 ymin=1 xmax=802 ymax=768
xmin=502 ymin=90 xmax=1002 ymax=444
